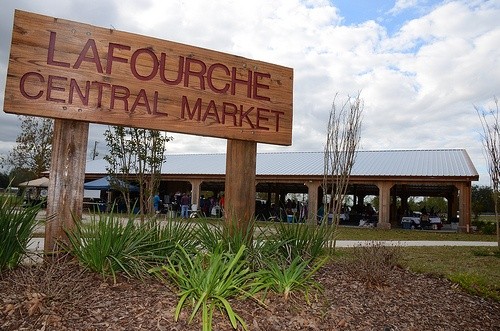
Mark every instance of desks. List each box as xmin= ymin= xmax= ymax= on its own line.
xmin=187 ymin=209 xmax=200 ymax=218
xmin=211 ymin=208 xmax=221 ymax=218
xmin=426 ymin=217 xmax=443 ymax=229
xmin=401 ymin=217 xmax=420 ymax=229
xmin=83 ymin=202 xmax=118 ymax=205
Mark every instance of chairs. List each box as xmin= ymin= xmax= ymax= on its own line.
xmin=268 ymin=211 xmax=278 ymax=219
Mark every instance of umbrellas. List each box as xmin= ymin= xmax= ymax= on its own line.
xmin=20 ymin=176 xmax=49 ymax=187
xmin=84 ymin=175 xmax=138 ymax=202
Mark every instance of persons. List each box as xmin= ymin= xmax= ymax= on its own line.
xmin=421 ymin=212 xmax=430 ymax=229
xmin=391 ymin=204 xmax=408 ymax=227
xmin=180 ymin=193 xmax=189 ymax=219
xmin=200 ymin=192 xmax=224 ymax=217
xmin=431 ymin=207 xmax=434 ymax=216
xmin=273 ymin=204 xmax=278 ymax=217
xmin=421 ymin=206 xmax=426 ymax=213
xmin=287 ymin=199 xmax=300 ymax=213
xmin=154 ymin=192 xmax=162 ymax=211
xmin=171 ymin=191 xmax=182 ymax=210
